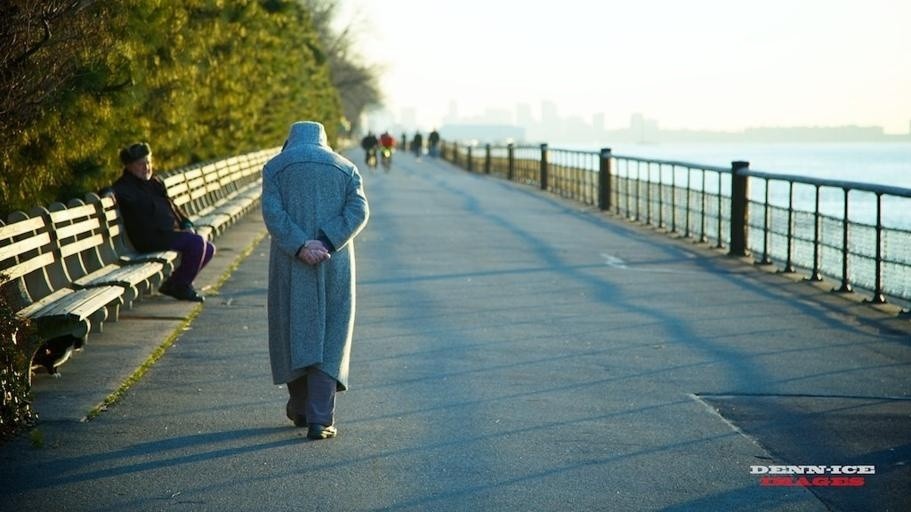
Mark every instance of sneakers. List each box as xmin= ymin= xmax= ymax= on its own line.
xmin=159 ymin=279 xmax=205 ymax=301
xmin=286 ymin=399 xmax=307 ymax=427
xmin=307 ymin=424 xmax=338 ymax=439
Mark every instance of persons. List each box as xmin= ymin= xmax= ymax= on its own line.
xmin=110 ymin=140 xmax=216 ymax=303
xmin=362 ymin=128 xmax=378 ymax=158
xmin=262 ymin=119 xmax=372 ymax=443
xmin=428 ymin=129 xmax=441 ymax=156
xmin=378 ymin=131 xmax=395 ymax=154
xmin=413 ymin=130 xmax=423 ymax=158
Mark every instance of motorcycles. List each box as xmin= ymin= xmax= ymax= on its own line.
xmin=366 ymin=148 xmax=378 ymax=169
xmin=380 ymin=149 xmax=396 ymax=172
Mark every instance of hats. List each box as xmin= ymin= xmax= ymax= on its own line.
xmin=120 ymin=142 xmax=151 ymax=164
